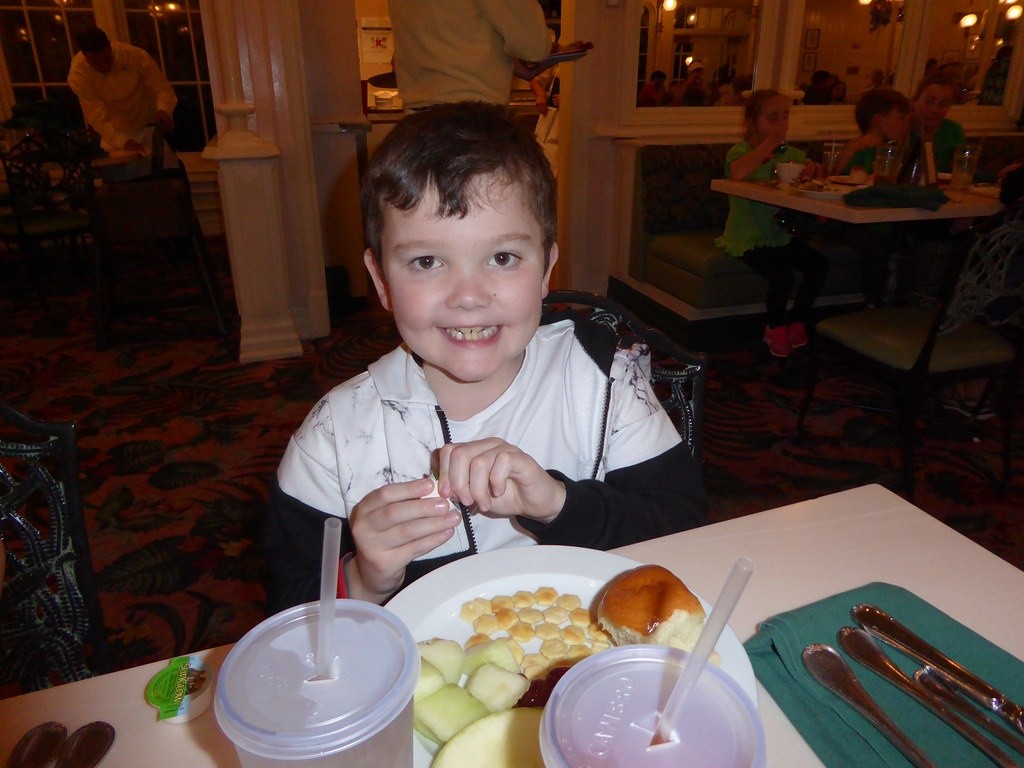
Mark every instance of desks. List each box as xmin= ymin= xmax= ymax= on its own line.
xmin=0 ymin=472 xmax=1024 ymax=768
xmin=702 ymin=160 xmax=1005 ymax=237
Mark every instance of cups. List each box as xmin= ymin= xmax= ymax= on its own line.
xmin=949 ymin=143 xmax=981 ymax=193
xmin=213 ymin=595 xmax=422 ymax=767
xmin=874 ymin=145 xmax=905 ymax=184
xmin=539 ymin=645 xmax=768 ymax=768
xmin=823 ymin=143 xmax=846 ymax=175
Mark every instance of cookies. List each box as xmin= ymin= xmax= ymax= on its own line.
xmin=460 ymin=587 xmax=614 ymax=679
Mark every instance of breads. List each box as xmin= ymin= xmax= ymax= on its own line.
xmin=850 ymin=166 xmax=868 ymax=183
xmin=599 ymin=564 xmax=719 ymax=664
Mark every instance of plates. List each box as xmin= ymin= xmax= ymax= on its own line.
xmin=521 ymin=48 xmax=585 ymax=63
xmin=970 ymin=182 xmax=1001 ymax=198
xmin=797 ymin=183 xmax=854 ymax=199
xmin=937 ymin=172 xmax=952 ymax=181
xmin=381 ymin=544 xmax=756 ymax=768
xmin=828 ymin=175 xmax=866 ymax=184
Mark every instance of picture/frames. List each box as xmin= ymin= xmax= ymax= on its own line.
xmin=806 ymin=29 xmax=820 ymax=49
xmin=802 ymin=52 xmax=817 ymax=70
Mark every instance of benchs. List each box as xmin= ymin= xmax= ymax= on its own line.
xmin=611 ymin=136 xmax=882 ymax=323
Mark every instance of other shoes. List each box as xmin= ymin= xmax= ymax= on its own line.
xmin=942 ymin=386 xmax=996 ymax=420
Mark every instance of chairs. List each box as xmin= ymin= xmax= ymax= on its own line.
xmin=0 ymin=389 xmax=111 ymax=699
xmin=0 ymin=125 xmax=104 ymax=300
xmin=802 ymin=210 xmax=1024 ymax=490
xmin=96 ymin=117 xmax=231 ymax=352
xmin=533 ymin=288 xmax=712 ymax=466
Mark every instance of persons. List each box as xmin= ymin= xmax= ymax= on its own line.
xmin=810 ymin=88 xmax=910 ymax=314
xmin=636 ymin=42 xmax=1017 ymax=107
xmin=905 ymin=66 xmax=965 ymax=250
xmin=252 ymin=99 xmax=707 ymax=639
xmin=385 ymin=0 xmax=595 ymax=136
xmin=715 ymin=89 xmax=825 ymax=359
xmin=67 ymin=26 xmax=222 ymax=305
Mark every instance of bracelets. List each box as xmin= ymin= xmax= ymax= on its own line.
xmin=337 ymin=552 xmax=358 ymax=600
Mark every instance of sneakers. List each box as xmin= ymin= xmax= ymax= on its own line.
xmin=791 ymin=322 xmax=808 ymax=348
xmin=763 ymin=325 xmax=795 ymax=357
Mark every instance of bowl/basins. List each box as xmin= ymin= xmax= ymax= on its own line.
xmin=775 ymin=163 xmax=805 ymax=181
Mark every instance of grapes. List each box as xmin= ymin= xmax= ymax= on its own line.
xmin=515 ymin=666 xmax=572 ymax=709
